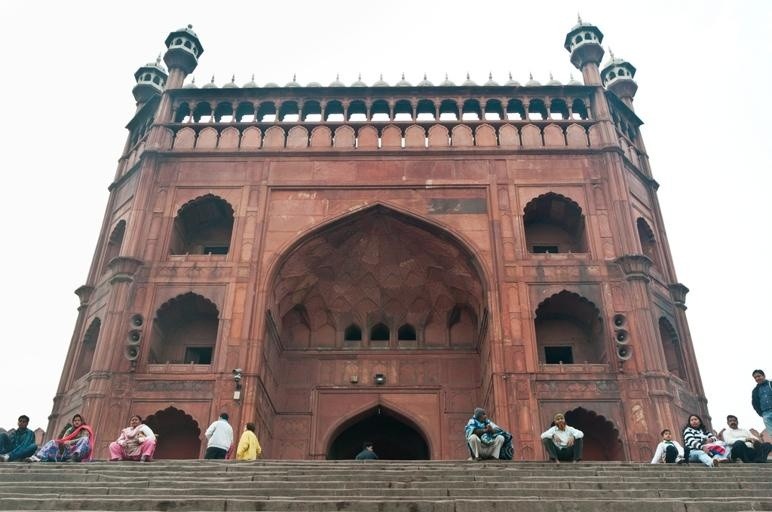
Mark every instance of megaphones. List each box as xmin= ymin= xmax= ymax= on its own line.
xmin=124 ymin=344 xmax=140 ymax=361
xmin=126 ymin=328 xmax=142 ymax=345
xmin=611 ymin=312 xmax=629 ymax=330
xmin=615 ymin=344 xmax=633 ymax=360
xmin=129 ymin=313 xmax=145 ymax=329
xmin=613 ymin=328 xmax=632 ymax=345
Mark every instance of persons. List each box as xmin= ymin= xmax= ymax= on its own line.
xmin=1 ymin=415 xmax=37 ymax=461
xmin=235 ymin=422 xmax=263 ymax=459
xmin=26 ymin=413 xmax=95 ymax=462
xmin=110 ymin=414 xmax=157 ymax=461
xmin=355 ymin=440 xmax=380 ymax=459
xmin=683 ymin=414 xmax=731 ymax=467
xmin=724 ymin=414 xmax=772 ymax=462
xmin=751 ymin=369 xmax=772 ymax=438
xmin=465 ymin=407 xmax=514 ymax=460
xmin=204 ymin=412 xmax=234 ymax=458
xmin=649 ymin=429 xmax=685 ymax=464
xmin=541 ymin=412 xmax=584 ymax=464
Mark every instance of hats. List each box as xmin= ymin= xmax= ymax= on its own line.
xmin=475 ymin=408 xmax=485 ymax=418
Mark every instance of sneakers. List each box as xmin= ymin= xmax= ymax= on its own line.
xmin=712 ymin=457 xmax=718 ymax=466
xmin=674 ymin=456 xmax=685 ymax=463
xmin=72 ymin=450 xmax=81 ymax=462
xmin=25 ymin=455 xmax=41 ymax=462
xmin=0 ymin=454 xmax=9 ymax=462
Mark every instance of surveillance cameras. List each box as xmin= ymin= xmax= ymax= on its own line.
xmin=233 ymin=375 xmax=241 ymax=382
xmin=232 ymin=368 xmax=242 ymax=373
